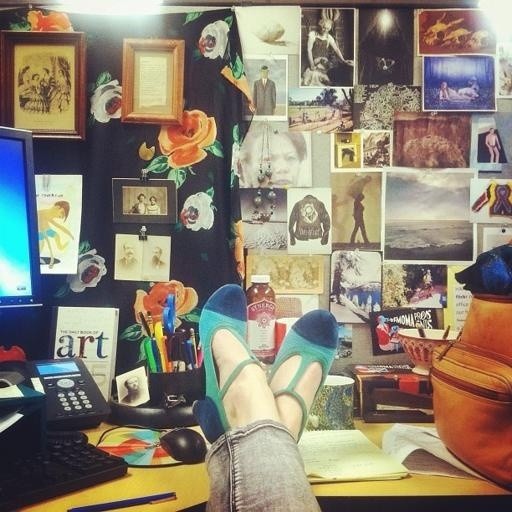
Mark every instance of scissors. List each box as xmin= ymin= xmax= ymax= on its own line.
xmin=163 ymin=294 xmax=176 ymax=332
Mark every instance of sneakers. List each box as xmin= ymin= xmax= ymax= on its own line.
xmin=268 ymin=308 xmax=338 ymax=443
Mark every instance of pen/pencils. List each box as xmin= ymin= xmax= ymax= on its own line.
xmin=139 ymin=311 xmax=203 ymax=373
xmin=456 ymin=328 xmax=463 ymax=340
xmin=442 ymin=325 xmax=450 ymax=338
xmin=417 ymin=323 xmax=426 ymax=337
xmin=66 ymin=492 xmax=176 ymax=512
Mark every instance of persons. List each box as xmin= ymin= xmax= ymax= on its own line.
xmin=485 ymin=127 xmax=502 ymax=163
xmin=438 ymin=81 xmax=479 ymax=101
xmin=189 ymin=284 xmax=338 ymax=512
xmin=302 ymin=8 xmax=354 ymax=86
xmin=118 ymin=243 xmax=138 ymax=269
xmin=128 ymin=194 xmax=162 ymax=216
xmin=350 ymin=193 xmax=372 ymax=247
xmin=148 ymin=247 xmax=168 ymax=269
xmin=18 ymin=63 xmax=71 ymax=112
xmin=376 ymin=315 xmax=397 ymax=351
xmin=237 ymin=123 xmax=311 ymax=185
xmin=123 ymin=378 xmax=146 ymax=405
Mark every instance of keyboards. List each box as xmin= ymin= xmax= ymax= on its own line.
xmin=0 ymin=443 xmax=127 ymax=511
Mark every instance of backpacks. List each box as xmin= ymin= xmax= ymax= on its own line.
xmin=430 ymin=291 xmax=512 ymax=489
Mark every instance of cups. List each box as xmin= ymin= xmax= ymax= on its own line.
xmin=304 ymin=374 xmax=355 ymax=430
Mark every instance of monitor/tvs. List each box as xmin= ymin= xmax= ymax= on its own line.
xmin=0 ymin=127 xmax=46 ymax=387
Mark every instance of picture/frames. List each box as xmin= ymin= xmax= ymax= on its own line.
xmin=111 ymin=176 xmax=181 ymax=227
xmin=120 ymin=37 xmax=185 ymax=126
xmin=1 ymin=30 xmax=88 ymax=142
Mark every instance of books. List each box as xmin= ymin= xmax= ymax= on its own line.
xmin=293 ymin=427 xmax=409 ymax=483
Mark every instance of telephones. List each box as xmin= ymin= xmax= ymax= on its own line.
xmin=0 ymin=358 xmax=111 ymax=430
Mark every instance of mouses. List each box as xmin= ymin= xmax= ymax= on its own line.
xmin=159 ymin=428 xmax=207 ymax=464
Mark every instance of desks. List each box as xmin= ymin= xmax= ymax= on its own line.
xmin=14 ymin=388 xmax=510 ymax=512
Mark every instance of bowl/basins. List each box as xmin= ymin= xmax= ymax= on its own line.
xmin=397 ymin=326 xmax=463 ymax=376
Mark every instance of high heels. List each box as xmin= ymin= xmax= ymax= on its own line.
xmin=191 ymin=284 xmax=266 ymax=443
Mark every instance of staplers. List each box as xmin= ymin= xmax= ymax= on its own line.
xmin=363 ymin=388 xmax=434 ymax=423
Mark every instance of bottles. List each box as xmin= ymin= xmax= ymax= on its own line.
xmin=245 ymin=272 xmax=277 ymax=363
